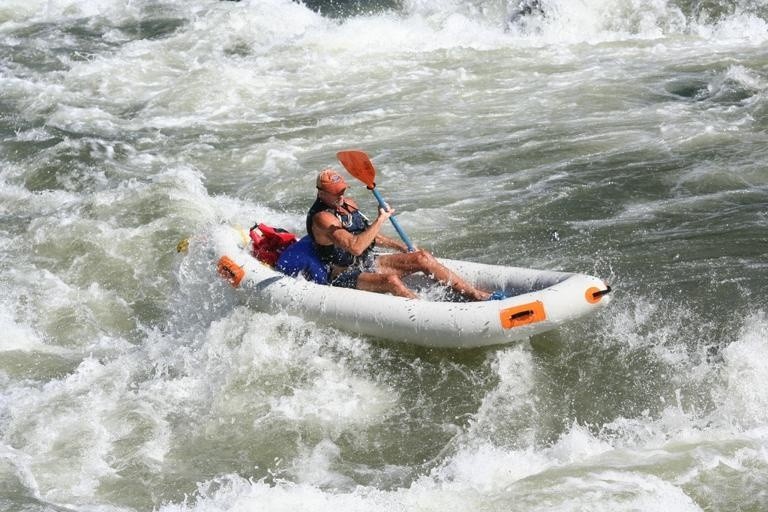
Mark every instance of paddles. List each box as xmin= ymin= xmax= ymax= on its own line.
xmin=335 ymin=151 xmax=416 ymax=253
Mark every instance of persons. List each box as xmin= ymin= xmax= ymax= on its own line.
xmin=305 ymin=168 xmax=508 ymax=302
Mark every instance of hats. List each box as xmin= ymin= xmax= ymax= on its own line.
xmin=316 ymin=168 xmax=351 ymax=194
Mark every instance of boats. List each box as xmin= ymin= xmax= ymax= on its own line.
xmin=216 ymin=228 xmax=612 ymax=348
